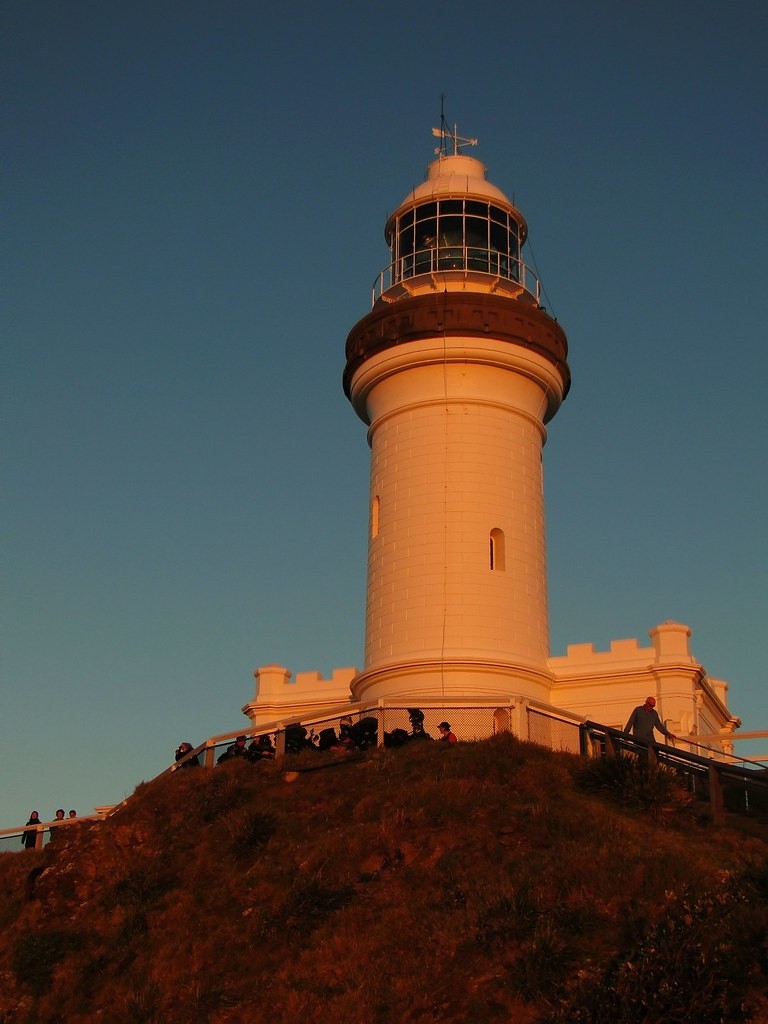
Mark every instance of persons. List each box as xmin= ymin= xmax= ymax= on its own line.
xmin=174 ymin=743 xmax=200 ymax=768
xmin=437 ymin=722 xmax=457 ymax=742
xmin=225 ymin=709 xmax=432 ymax=763
xmin=624 ymin=697 xmax=677 ymax=769
xmin=49 ymin=809 xmax=65 ymax=841
xmin=66 ymin=810 xmax=77 ymax=819
xmin=21 ymin=811 xmax=42 ymax=849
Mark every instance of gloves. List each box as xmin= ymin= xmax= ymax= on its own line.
xmin=21 ymin=841 xmax=24 ymax=844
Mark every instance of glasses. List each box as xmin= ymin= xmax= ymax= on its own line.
xmin=648 ymin=702 xmax=655 ymax=707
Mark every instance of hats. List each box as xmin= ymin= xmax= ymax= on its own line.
xmin=237 ymin=736 xmax=246 ymax=740
xmin=437 ymin=722 xmax=451 ymax=728
